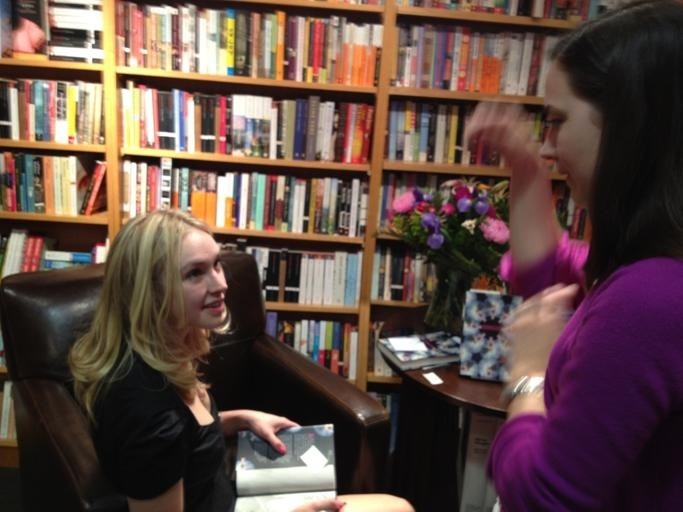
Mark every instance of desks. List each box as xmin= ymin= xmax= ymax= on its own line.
xmin=378 ymin=312 xmax=518 ymax=511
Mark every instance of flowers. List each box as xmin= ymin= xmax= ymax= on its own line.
xmin=390 ymin=177 xmax=568 ymax=329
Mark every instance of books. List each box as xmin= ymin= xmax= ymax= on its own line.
xmin=233 ymin=420 xmax=337 ymax=512
xmin=1 ymin=1 xmax=109 ymax=278
xmin=379 ymin=331 xmax=462 ymax=371
xmin=115 ymin=0 xmax=384 ymax=381
xmin=384 ymin=2 xmax=590 ymax=303
xmin=459 ymin=291 xmax=524 ymax=384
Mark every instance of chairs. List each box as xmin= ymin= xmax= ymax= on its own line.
xmin=0 ymin=250 xmax=391 ymax=512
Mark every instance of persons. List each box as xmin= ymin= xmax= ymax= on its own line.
xmin=69 ymin=208 xmax=415 ymax=512
xmin=462 ymin=1 xmax=683 ymax=510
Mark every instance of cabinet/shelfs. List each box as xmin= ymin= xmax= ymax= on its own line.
xmin=0 ymin=0 xmax=591 ymax=397
